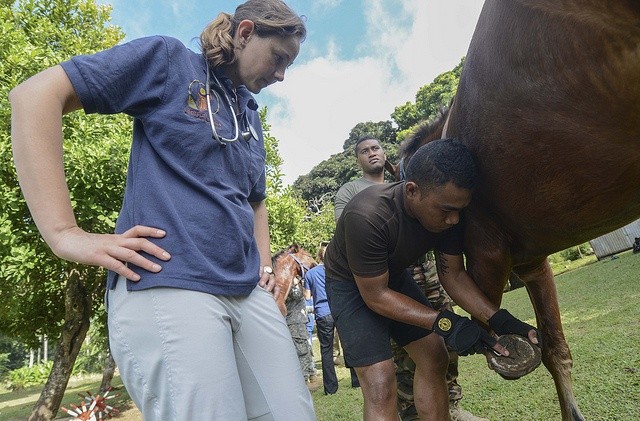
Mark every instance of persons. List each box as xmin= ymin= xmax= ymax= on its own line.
xmin=286 ymin=278 xmax=315 ymax=384
xmin=334 ymin=135 xmax=491 ymax=421
xmin=324 ymin=137 xmax=539 ymax=421
xmin=301 ymin=246 xmax=360 ymax=397
xmin=9 ymin=0 xmax=315 ymax=421
xmin=303 ymin=296 xmax=314 ymax=346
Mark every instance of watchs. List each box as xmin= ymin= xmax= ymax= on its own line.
xmin=263 ymin=265 xmax=275 ymax=276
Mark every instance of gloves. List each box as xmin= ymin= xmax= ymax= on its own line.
xmin=432 ymin=309 xmax=497 ymax=356
xmin=487 ymin=308 xmax=538 ymax=338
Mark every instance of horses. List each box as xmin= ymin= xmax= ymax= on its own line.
xmin=384 ymin=0 xmax=640 ymax=420
xmin=270 ymin=241 xmax=319 ymax=318
